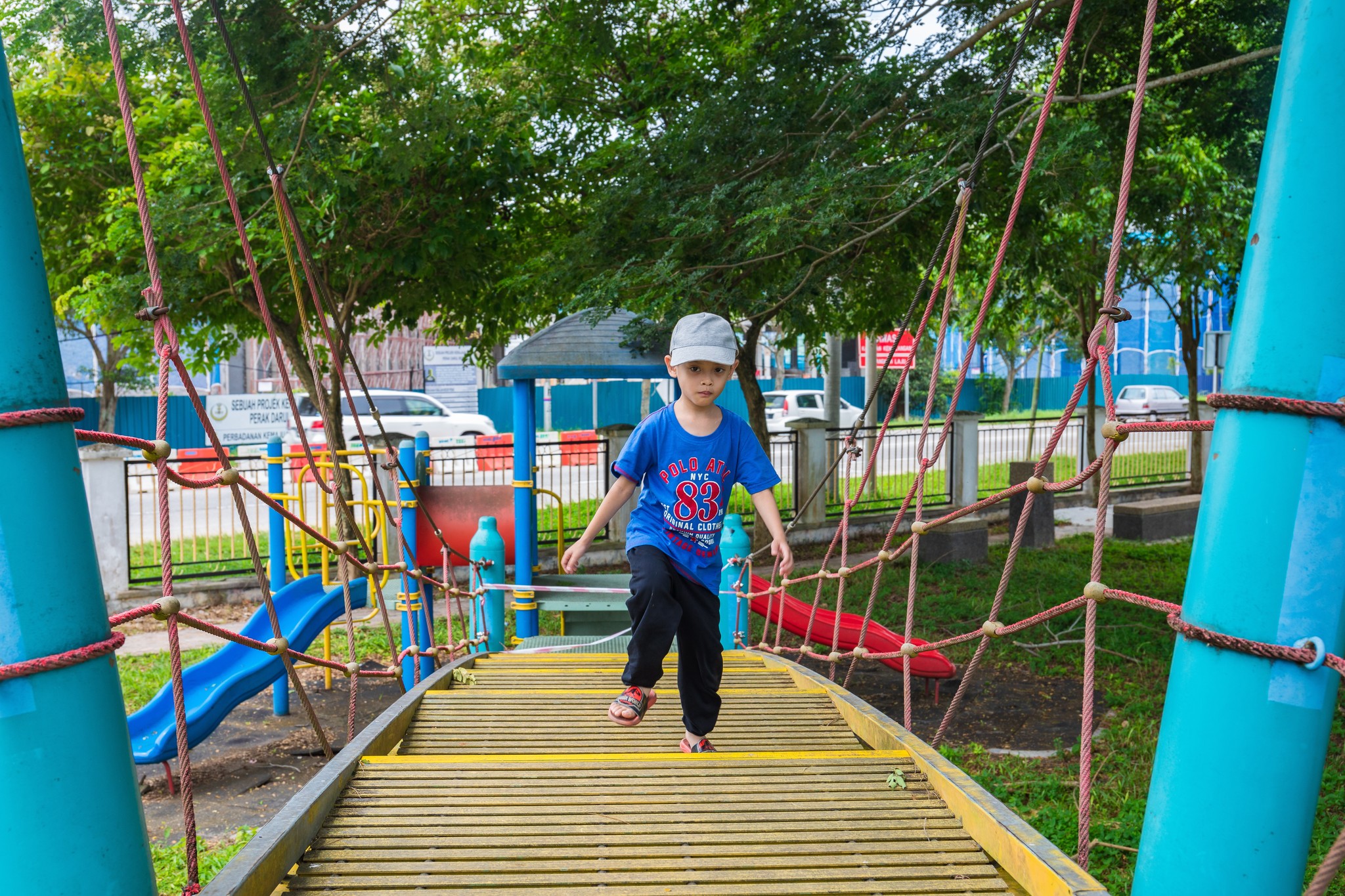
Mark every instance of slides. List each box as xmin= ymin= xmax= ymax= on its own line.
xmin=747 ymin=573 xmax=956 ymax=680
xmin=126 ymin=573 xmax=368 ymax=766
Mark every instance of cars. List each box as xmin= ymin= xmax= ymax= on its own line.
xmin=1116 ymin=385 xmax=1190 ymax=422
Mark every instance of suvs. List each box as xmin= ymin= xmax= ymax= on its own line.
xmin=290 ymin=389 xmax=500 ymax=452
xmin=760 ymin=390 xmax=866 ymax=432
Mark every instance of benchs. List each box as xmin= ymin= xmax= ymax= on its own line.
xmin=1114 ymin=494 xmax=1201 ymax=541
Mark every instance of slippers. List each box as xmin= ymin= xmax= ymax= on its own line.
xmin=607 ymin=685 xmax=657 ymax=726
xmin=679 ymin=737 xmax=717 ymax=753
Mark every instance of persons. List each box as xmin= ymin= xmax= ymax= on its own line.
xmin=561 ymin=313 xmax=794 ymax=756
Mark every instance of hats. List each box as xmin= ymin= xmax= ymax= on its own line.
xmin=669 ymin=311 xmax=739 ymax=367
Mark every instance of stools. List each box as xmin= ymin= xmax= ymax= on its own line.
xmin=918 ymin=516 xmax=988 ymax=562
